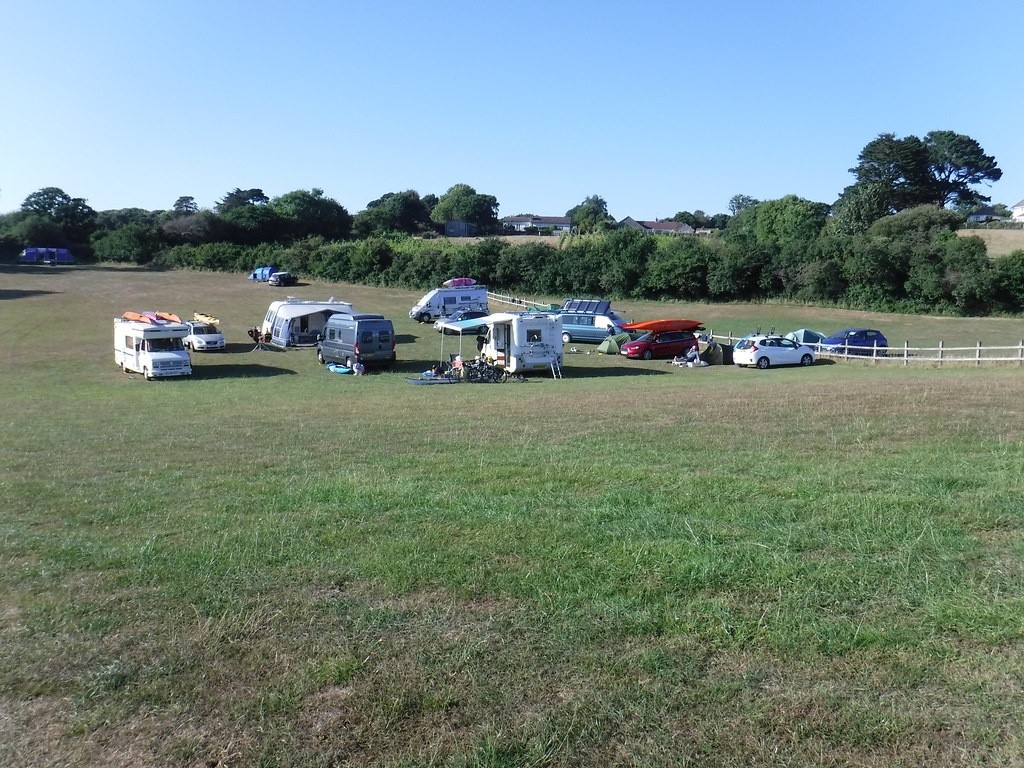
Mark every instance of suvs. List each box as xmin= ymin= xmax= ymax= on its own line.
xmin=181 ymin=320 xmax=226 ymax=352
xmin=733 ymin=334 xmax=815 ymax=370
xmin=434 ymin=309 xmax=489 ymax=335
xmin=815 ymin=328 xmax=889 ymax=356
xmin=620 ymin=329 xmax=699 ymax=360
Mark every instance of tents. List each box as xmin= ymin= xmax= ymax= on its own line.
xmin=701 ymin=341 xmax=736 ymax=365
xmin=18 ymin=247 xmax=74 ymax=265
xmin=248 ymin=267 xmax=276 ymax=283
xmin=527 ymin=303 xmax=561 ymax=313
xmin=780 ymin=329 xmax=828 ymax=351
xmin=597 ymin=332 xmax=646 ymax=355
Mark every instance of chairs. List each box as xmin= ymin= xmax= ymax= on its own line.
xmin=769 ymin=341 xmax=774 ymax=346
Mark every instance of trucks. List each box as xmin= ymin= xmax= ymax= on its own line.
xmin=114 ymin=317 xmax=192 ymax=380
xmin=409 ymin=285 xmax=488 ymax=323
xmin=476 ymin=310 xmax=564 ymax=378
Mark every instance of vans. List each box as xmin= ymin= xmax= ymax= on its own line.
xmin=558 ymin=312 xmax=637 ymax=343
xmin=269 ymin=272 xmax=292 ymax=286
xmin=317 ymin=314 xmax=396 ymax=368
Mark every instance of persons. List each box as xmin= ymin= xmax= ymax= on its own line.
xmin=687 ymin=345 xmax=701 ymax=363
xmin=607 ymin=324 xmax=616 ymax=337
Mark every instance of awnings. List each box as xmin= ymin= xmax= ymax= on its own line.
xmin=436 ymin=314 xmax=512 ymax=370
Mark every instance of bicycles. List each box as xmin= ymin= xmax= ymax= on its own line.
xmin=448 ymin=357 xmax=507 ymax=383
